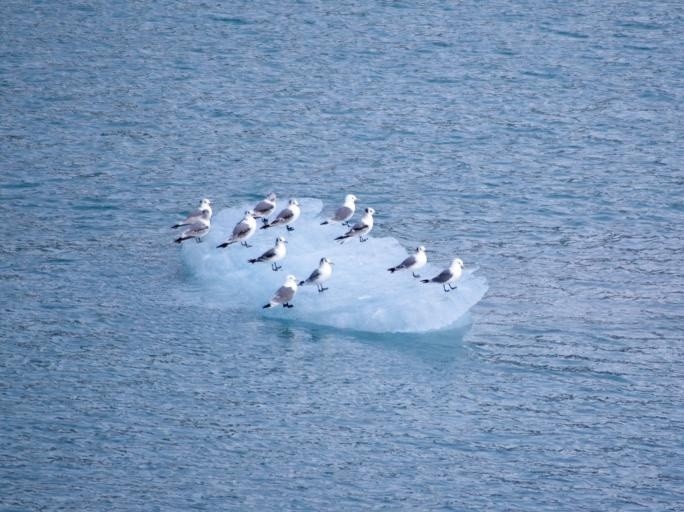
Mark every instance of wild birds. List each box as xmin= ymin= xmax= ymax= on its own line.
xmin=420 ymin=257 xmax=464 ymax=291
xmin=387 ymin=245 xmax=428 ymax=279
xmin=261 ymin=274 xmax=298 ymax=310
xmin=320 ymin=193 xmax=360 ymax=227
xmin=334 ymin=206 xmax=375 ymax=245
xmin=247 ymin=234 xmax=289 ymax=271
xmin=169 ymin=197 xmax=213 ymax=245
xmin=216 ymin=207 xmax=257 ymax=249
xmin=250 ymin=191 xmax=277 ymax=223
xmin=299 ymin=257 xmax=334 ymax=293
xmin=259 ymin=198 xmax=302 ymax=233
